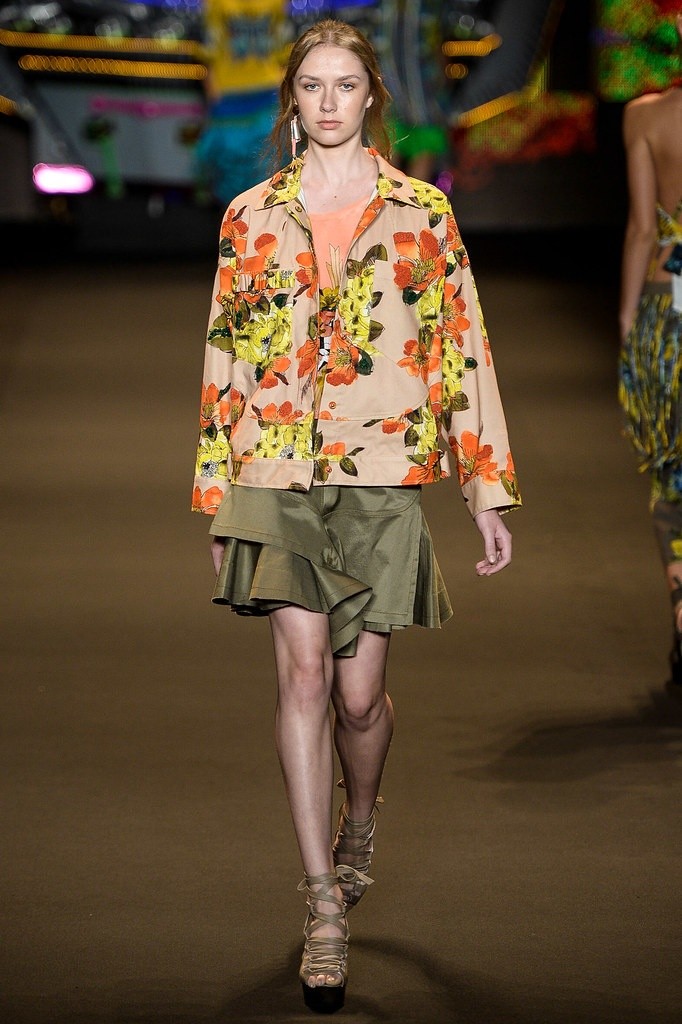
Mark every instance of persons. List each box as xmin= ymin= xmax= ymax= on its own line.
xmin=615 ymin=84 xmax=682 ymax=712
xmin=191 ymin=19 xmax=524 ymax=1017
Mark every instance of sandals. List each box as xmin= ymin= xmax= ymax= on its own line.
xmin=332 ymin=778 xmax=384 ymax=915
xmin=300 ymin=864 xmax=376 ymax=989
